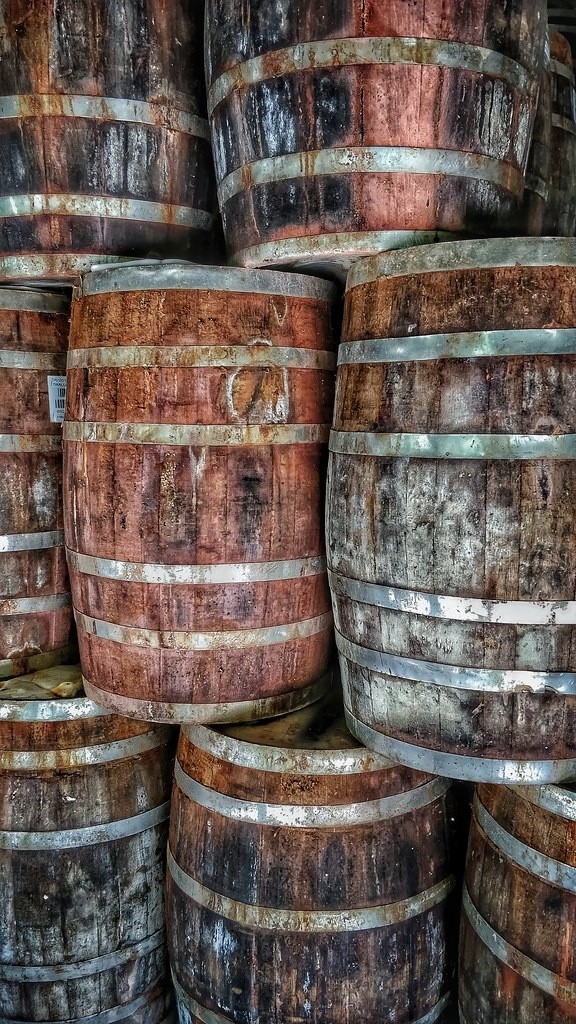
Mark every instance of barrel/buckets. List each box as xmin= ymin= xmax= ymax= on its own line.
xmin=510 ymin=28 xmax=550 ymax=237
xmin=0 ymin=290 xmax=81 ymax=676
xmin=324 ymin=237 xmax=576 ymax=784
xmin=0 ymin=700 xmax=172 ymax=1024
xmin=460 ymin=782 xmax=576 ymax=1024
xmin=207 ymin=0 xmax=544 ymax=281
xmin=63 ymin=264 xmax=341 ymax=725
xmin=549 ymin=31 xmax=576 ymax=236
xmin=0 ymin=0 xmax=221 ymax=283
xmin=166 ymin=699 xmax=461 ymax=1024
